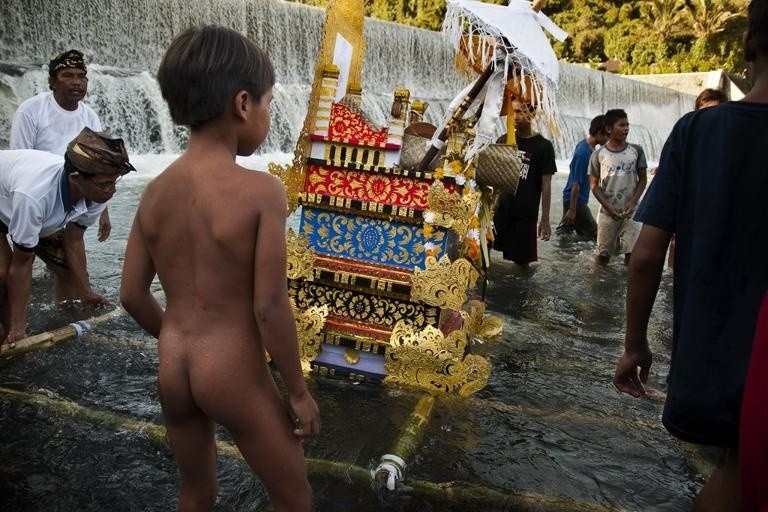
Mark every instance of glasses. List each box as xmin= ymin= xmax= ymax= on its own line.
xmin=90 ymin=175 xmax=123 ymax=190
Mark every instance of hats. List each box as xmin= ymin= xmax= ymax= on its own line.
xmin=67 ymin=127 xmax=130 ymax=175
xmin=49 ymin=50 xmax=87 ymax=77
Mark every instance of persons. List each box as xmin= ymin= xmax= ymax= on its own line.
xmin=1 ymin=124 xmax=139 ymax=358
xmin=587 ymin=108 xmax=648 ymax=266
xmin=6 ymin=49 xmax=113 ymax=305
xmin=695 ymin=88 xmax=729 ymax=115
xmin=555 ymin=113 xmax=611 ymax=246
xmin=611 ymin=1 xmax=767 ymax=512
xmin=117 ymin=26 xmax=326 ymax=512
xmin=486 ymin=94 xmax=557 ymax=273
